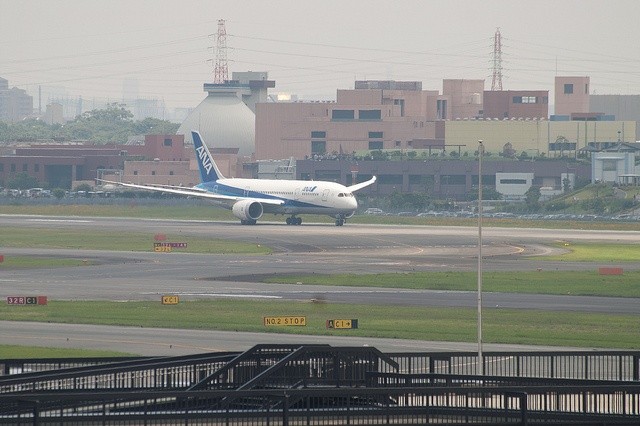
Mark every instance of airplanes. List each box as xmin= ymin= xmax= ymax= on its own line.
xmin=94 ymin=131 xmax=376 ymax=226
